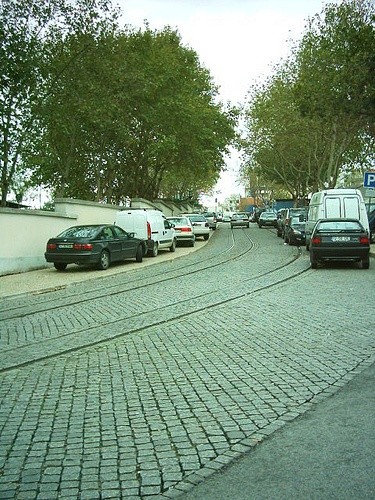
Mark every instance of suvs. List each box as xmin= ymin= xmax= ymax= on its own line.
xmin=277 ymin=209 xmax=285 ymax=237
xmin=200 ymin=212 xmax=217 ymax=231
xmin=178 ymin=214 xmax=210 ymax=240
xmin=251 ymin=208 xmax=266 ymax=222
xmin=283 ymin=208 xmax=308 ymax=239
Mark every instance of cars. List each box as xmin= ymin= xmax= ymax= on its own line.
xmin=166 ymin=216 xmax=195 ymax=247
xmin=230 ymin=213 xmax=249 ymax=229
xmin=284 ymin=217 xmax=306 ymax=245
xmin=306 ymin=218 xmax=370 ymax=269
xmin=258 ymin=212 xmax=278 ymax=228
xmin=45 ymin=224 xmax=146 ymax=270
xmin=249 ymin=215 xmax=255 ymax=222
xmin=365 ymin=202 xmax=375 ymax=244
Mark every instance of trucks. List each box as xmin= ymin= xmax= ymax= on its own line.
xmin=216 ymin=212 xmax=237 ymax=222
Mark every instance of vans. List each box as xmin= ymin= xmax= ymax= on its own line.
xmin=305 ymin=189 xmax=370 ymax=252
xmin=113 ymin=208 xmax=177 ymax=257
